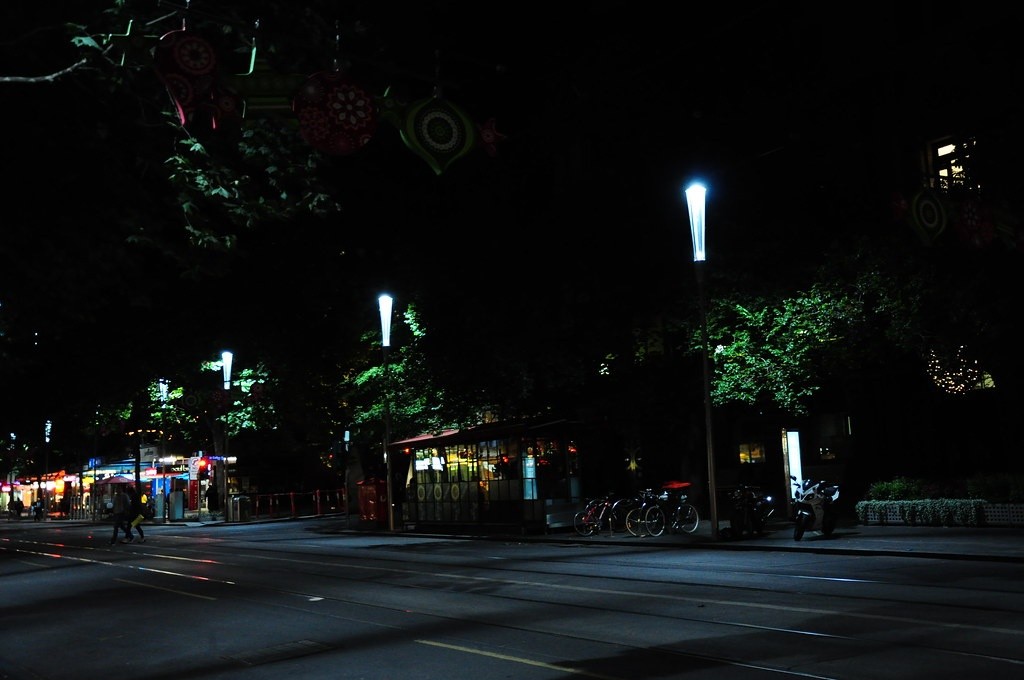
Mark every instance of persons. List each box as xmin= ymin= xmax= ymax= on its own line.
xmin=794 ymin=441 xmax=837 ymax=535
xmin=104 ymin=485 xmax=146 ymax=547
xmin=204 ymin=478 xmax=220 ymax=521
xmin=160 ymin=488 xmax=185 ymax=523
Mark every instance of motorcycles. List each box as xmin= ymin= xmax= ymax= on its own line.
xmin=793 ymin=479 xmax=843 ymax=542
xmin=727 ymin=481 xmax=777 ymax=539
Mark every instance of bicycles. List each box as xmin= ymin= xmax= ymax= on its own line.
xmin=574 ymin=488 xmax=699 ymax=537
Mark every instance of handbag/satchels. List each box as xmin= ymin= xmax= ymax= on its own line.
xmin=130 ymin=512 xmax=144 ymax=528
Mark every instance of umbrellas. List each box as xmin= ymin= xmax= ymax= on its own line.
xmin=93 ymin=475 xmax=134 ymax=484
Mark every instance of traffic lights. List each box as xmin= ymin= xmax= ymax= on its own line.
xmin=198 ymin=457 xmax=207 ymax=471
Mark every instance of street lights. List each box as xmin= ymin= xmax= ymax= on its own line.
xmin=376 ymin=293 xmax=394 ymax=531
xmin=222 ymin=350 xmax=234 ymax=523
xmin=157 ymin=378 xmax=169 ymax=525
xmin=43 ymin=419 xmax=52 ymax=520
xmin=684 ymin=181 xmax=719 ymax=542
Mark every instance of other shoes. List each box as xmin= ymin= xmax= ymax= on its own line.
xmin=121 ymin=537 xmax=130 ymax=543
xmin=129 ymin=537 xmax=135 ymax=544
xmin=106 ymin=541 xmax=115 ymax=547
xmin=137 ymin=537 xmax=145 ymax=544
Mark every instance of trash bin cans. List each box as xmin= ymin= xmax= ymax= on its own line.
xmin=227 ymin=491 xmax=252 ymax=522
